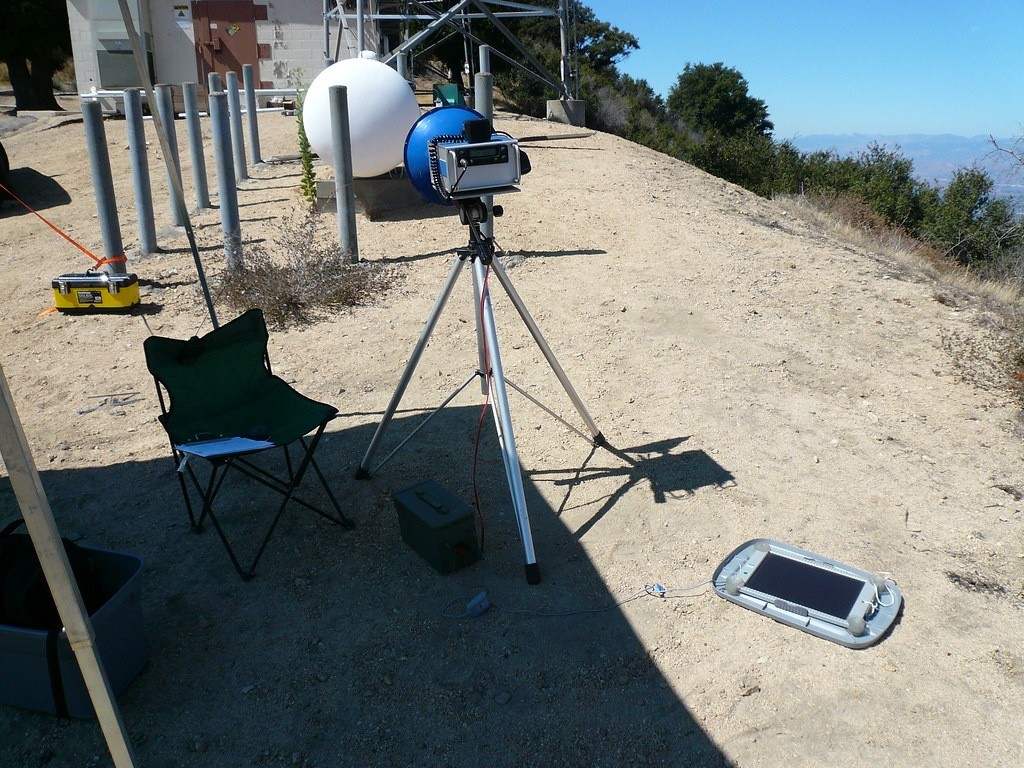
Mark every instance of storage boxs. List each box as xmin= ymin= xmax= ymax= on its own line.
xmin=391 ymin=480 xmax=480 ymax=576
xmin=0 ymin=530 xmax=144 ymax=717
xmin=52 ymin=270 xmax=141 ymax=313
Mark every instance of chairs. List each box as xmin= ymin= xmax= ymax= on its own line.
xmin=142 ymin=308 xmax=355 ymax=583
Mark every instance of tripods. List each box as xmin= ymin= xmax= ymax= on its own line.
xmin=350 ymin=207 xmax=609 ymax=583
